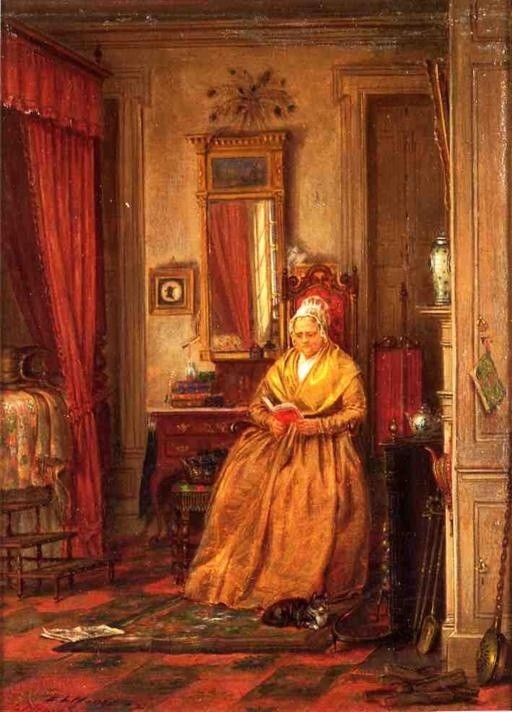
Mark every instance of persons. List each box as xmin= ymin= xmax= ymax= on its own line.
xmin=180 ymin=296 xmax=373 ymax=612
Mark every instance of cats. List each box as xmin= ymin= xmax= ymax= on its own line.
xmin=261 ymin=590 xmax=331 ymax=632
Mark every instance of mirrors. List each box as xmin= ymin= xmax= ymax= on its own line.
xmin=186 ymin=129 xmax=288 ymax=361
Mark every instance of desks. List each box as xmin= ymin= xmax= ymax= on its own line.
xmin=378 ymin=436 xmax=444 ymax=648
xmin=146 ymin=406 xmax=254 ymax=548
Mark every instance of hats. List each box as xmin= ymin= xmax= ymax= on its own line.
xmin=288 ymin=294 xmax=335 ymax=355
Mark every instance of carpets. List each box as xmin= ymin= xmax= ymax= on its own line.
xmin=52 ymin=593 xmax=350 ymax=654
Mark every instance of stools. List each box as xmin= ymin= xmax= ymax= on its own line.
xmin=168 ymin=479 xmax=217 ymax=585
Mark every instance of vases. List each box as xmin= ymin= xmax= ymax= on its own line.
xmin=430 ymin=236 xmax=451 ymax=306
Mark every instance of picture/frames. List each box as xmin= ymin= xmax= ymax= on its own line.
xmin=150 ymin=267 xmax=196 ymax=315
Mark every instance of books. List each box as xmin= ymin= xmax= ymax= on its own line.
xmin=171 ymin=380 xmax=222 ymax=408
xmin=262 ymin=395 xmax=306 ymax=425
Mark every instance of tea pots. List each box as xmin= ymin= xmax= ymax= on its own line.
xmin=403 ymin=404 xmax=443 ymax=439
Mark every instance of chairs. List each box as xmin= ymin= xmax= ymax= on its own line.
xmin=230 ymin=262 xmax=371 ymax=467
